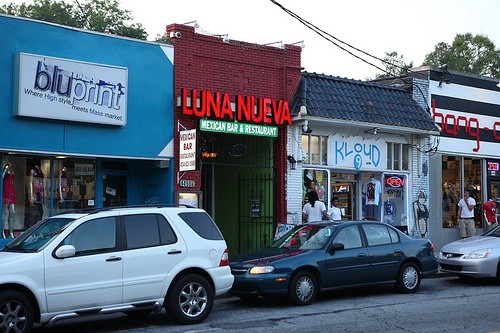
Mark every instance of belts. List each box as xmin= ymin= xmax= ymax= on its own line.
xmin=460 ymin=217 xmax=475 ymax=219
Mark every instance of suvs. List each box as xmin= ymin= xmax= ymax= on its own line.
xmin=0 ymin=203 xmax=235 ymax=333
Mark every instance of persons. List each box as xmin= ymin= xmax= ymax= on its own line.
xmin=57 ymin=166 xmax=75 ymax=213
xmin=483 ymin=199 xmax=498 ymax=231
xmin=326 ymin=197 xmax=351 ymax=221
xmin=2 ymin=162 xmax=15 ymax=239
xmin=28 ymin=165 xmax=45 ymax=229
xmin=456 ymin=188 xmax=476 ymax=238
xmin=301 ymin=191 xmax=333 ymax=223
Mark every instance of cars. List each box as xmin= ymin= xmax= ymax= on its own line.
xmin=228 ymin=221 xmax=439 ymax=306
xmin=437 ymin=222 xmax=500 ymax=280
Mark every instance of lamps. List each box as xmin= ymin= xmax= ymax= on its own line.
xmin=291 ymin=39 xmax=305 ymax=49
xmin=259 ymin=40 xmax=285 ymax=50
xmin=288 ymin=154 xmax=296 ymax=170
xmin=184 ymin=19 xmax=200 ymax=28
xmin=214 ymin=33 xmax=230 ymax=44
xmin=423 ymin=160 xmax=428 ymax=177
